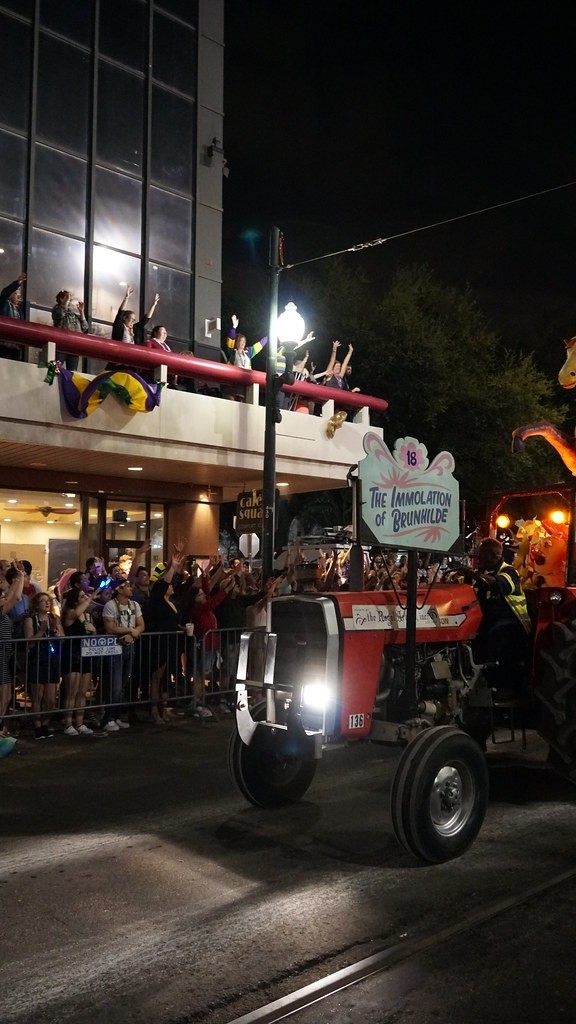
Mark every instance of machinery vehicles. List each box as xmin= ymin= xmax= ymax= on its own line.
xmin=226 ymin=492 xmax=576 ymax=867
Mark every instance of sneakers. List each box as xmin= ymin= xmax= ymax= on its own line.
xmin=76 ymin=724 xmax=93 ymax=735
xmin=102 ymin=721 xmax=119 ymax=731
xmin=64 ymin=726 xmax=78 ymax=736
xmin=116 ymin=719 xmax=130 ymax=729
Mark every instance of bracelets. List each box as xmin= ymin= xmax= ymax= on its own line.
xmin=16 ymin=577 xmax=20 ymax=582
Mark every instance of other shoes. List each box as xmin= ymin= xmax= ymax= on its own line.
xmin=43 ymin=727 xmax=55 ymax=738
xmin=216 ymin=701 xmax=230 ymax=713
xmin=34 ymin=729 xmax=45 ymax=740
xmin=193 ymin=706 xmax=211 ymax=717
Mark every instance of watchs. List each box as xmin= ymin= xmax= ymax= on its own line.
xmin=54 ymin=629 xmax=58 ymax=633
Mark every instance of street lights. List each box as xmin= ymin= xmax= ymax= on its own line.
xmin=252 ymin=229 xmax=305 ymax=710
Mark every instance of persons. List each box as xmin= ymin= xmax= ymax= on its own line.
xmin=106 ymin=284 xmax=175 ymax=388
xmin=224 ymin=315 xmax=361 ymax=423
xmin=268 ymin=539 xmax=478 ymax=598
xmin=51 ymin=290 xmax=89 ymax=374
xmin=0 ymin=536 xmax=276 ymax=745
xmin=0 ymin=273 xmax=27 ymax=361
xmin=468 ymin=537 xmax=532 ymax=702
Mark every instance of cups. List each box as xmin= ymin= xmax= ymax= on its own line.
xmin=186 ymin=623 xmax=194 ymax=637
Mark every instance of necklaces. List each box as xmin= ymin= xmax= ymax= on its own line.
xmin=153 ymin=338 xmax=171 ymax=353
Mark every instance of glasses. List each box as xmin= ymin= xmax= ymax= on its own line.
xmin=38 ymin=599 xmax=52 ymax=605
